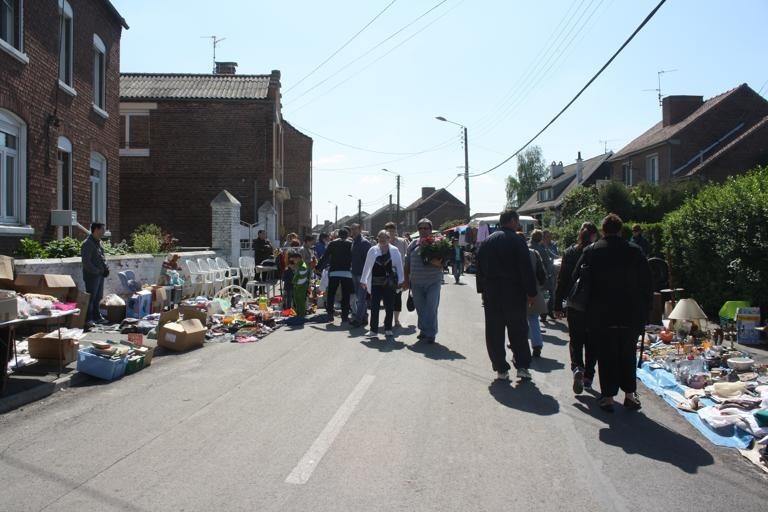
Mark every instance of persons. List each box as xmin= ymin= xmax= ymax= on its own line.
xmin=253 ymin=217 xmax=444 ymax=345
xmin=80 ymin=223 xmax=111 ymax=327
xmin=443 ymin=236 xmax=465 ymax=284
xmin=160 ymin=253 xmax=183 ymax=274
xmin=472 ymin=208 xmax=657 ymax=413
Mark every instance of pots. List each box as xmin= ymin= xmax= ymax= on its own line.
xmin=704 ymin=356 xmax=721 ymax=371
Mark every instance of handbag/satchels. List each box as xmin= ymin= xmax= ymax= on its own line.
xmin=407 ymin=296 xmax=415 ymax=312
xmin=383 ymin=272 xmax=396 ymax=290
xmin=533 ymin=250 xmax=545 ymax=285
xmin=567 ymin=242 xmax=609 ymax=313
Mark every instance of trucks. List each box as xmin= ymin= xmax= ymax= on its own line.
xmin=467 ymin=215 xmax=539 ymax=241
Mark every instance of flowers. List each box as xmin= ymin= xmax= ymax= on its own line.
xmin=416 ymin=232 xmax=453 ymax=262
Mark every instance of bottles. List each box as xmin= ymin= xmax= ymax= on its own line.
xmin=544 ymin=289 xmax=550 ymax=302
xmin=671 ymin=359 xmax=681 ymax=381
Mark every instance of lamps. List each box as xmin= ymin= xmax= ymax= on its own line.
xmin=669 ymin=297 xmax=708 ymax=346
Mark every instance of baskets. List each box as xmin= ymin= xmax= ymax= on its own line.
xmin=127 ymin=345 xmax=155 ymax=367
xmin=77 ymin=346 xmax=129 ymax=380
xmin=127 ymin=353 xmax=145 ymax=374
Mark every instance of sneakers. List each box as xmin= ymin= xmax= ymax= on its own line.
xmin=365 ymin=331 xmax=377 ymax=336
xmin=98 ymin=320 xmax=109 ymax=323
xmin=417 ymin=333 xmax=435 ymax=343
xmin=583 ymin=379 xmax=593 ymax=389
xmin=88 ymin=320 xmax=95 ymax=326
xmin=532 ymin=349 xmax=541 ymax=357
xmin=572 ymin=367 xmax=583 ymax=394
xmin=517 ymin=367 xmax=531 ymax=379
xmin=497 ymin=369 xmax=509 ymax=379
xmin=385 ymin=331 xmax=393 ymax=336
xmin=598 ymin=395 xmax=614 ymax=409
xmin=325 ymin=316 xmax=369 ymax=326
xmin=623 ymin=397 xmax=641 ymax=409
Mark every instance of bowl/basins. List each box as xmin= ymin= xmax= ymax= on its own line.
xmin=727 ymin=357 xmax=754 ymax=371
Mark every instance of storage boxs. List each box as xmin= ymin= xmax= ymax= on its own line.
xmin=0 ymin=251 xmax=209 ymax=381
xmin=734 ymin=305 xmax=762 ymax=346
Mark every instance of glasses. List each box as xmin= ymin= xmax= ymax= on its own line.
xmin=419 ymin=227 xmax=431 ymax=230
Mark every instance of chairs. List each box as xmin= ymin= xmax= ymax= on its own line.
xmin=179 ymin=255 xmax=272 ymax=299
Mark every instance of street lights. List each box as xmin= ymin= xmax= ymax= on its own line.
xmin=312 ymin=212 xmax=319 ymax=233
xmin=327 ymin=200 xmax=338 ymax=225
xmin=346 ymin=194 xmax=361 ymax=226
xmin=381 ymin=168 xmax=400 ymax=230
xmin=434 ymin=116 xmax=470 ymax=220
